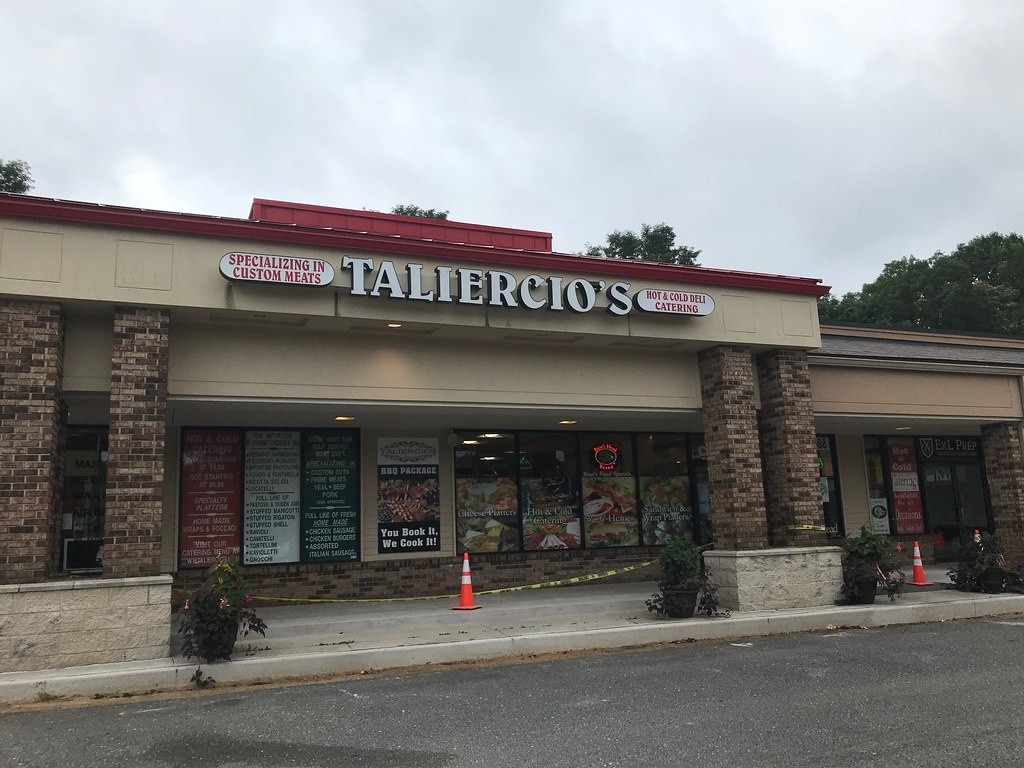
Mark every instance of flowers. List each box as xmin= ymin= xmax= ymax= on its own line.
xmin=175 ymin=558 xmax=269 ymax=687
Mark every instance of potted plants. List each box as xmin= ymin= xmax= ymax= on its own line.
xmin=834 ymin=527 xmax=906 ymax=607
xmin=946 ymin=529 xmax=1024 ymax=595
xmin=645 ymin=537 xmax=734 ymax=619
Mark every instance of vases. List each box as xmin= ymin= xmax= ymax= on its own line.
xmin=198 ymin=615 xmax=239 ymax=656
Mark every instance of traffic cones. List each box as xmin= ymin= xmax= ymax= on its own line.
xmin=905 ymin=540 xmax=935 ymax=585
xmin=451 ymin=551 xmax=483 ymax=610
xmin=971 ymin=529 xmax=985 ymax=553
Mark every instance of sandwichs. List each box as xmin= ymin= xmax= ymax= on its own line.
xmin=589 ymin=525 xmax=629 ymax=544
